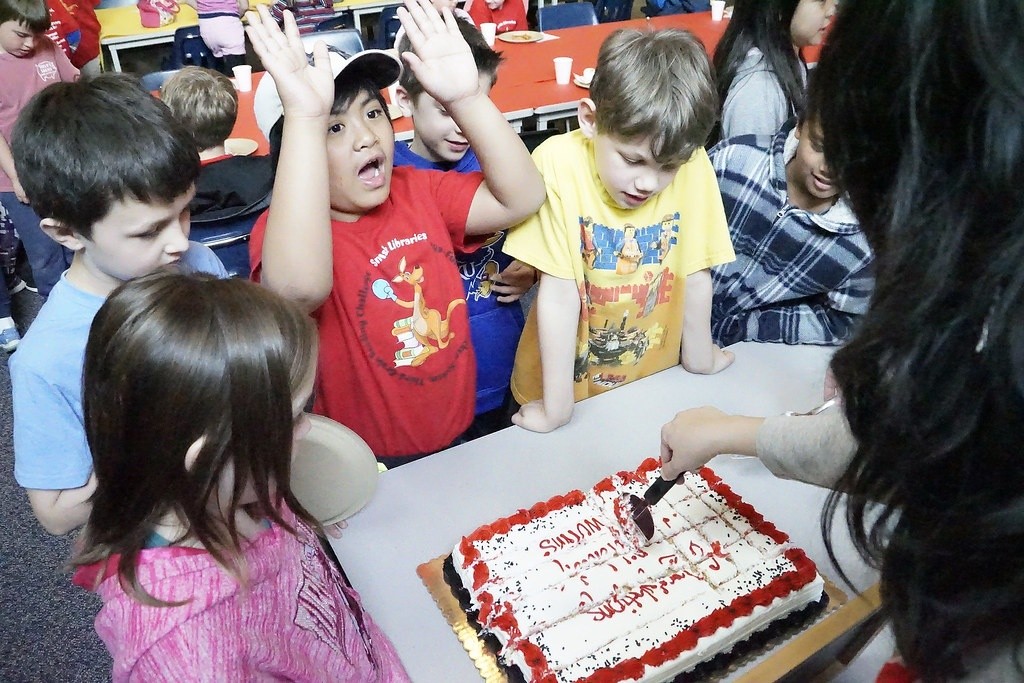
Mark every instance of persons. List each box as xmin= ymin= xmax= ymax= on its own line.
xmin=56 ymin=269 xmax=412 ymax=683
xmin=0 ymin=0 xmax=845 ymax=535
xmin=659 ymin=0 xmax=1024 ymax=683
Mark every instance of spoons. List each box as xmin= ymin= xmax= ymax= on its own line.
xmin=783 ymin=389 xmax=838 ymax=417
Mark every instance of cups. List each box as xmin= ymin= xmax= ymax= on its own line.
xmin=554 ymin=57 xmax=573 ymax=84
xmin=232 ymin=65 xmax=252 ymax=92
xmin=480 ymin=23 xmax=497 ymax=47
xmin=710 ymin=1 xmax=726 ymax=21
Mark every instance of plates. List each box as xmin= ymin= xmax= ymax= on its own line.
xmin=290 ymin=413 xmax=379 ymax=526
xmin=574 ymin=76 xmax=592 ymax=88
xmin=498 ymin=31 xmax=544 ymax=43
xmin=224 ymin=139 xmax=258 ymax=156
xmin=386 ymin=104 xmax=403 ymax=120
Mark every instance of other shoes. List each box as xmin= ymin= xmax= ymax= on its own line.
xmin=0 ymin=316 xmax=21 ymax=352
xmin=7 ymin=275 xmax=26 ymax=295
xmin=26 ymin=279 xmax=38 ymax=293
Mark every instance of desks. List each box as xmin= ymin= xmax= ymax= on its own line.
xmin=327 ymin=340 xmax=926 ymax=682
xmin=94 ymin=0 xmax=836 ymax=162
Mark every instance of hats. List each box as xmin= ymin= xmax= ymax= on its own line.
xmin=253 ymin=44 xmax=404 ymax=142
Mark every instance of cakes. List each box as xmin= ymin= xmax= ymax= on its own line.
xmin=442 ymin=455 xmax=832 ymax=683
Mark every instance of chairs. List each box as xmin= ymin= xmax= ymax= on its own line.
xmin=187 ymin=206 xmax=265 ymax=280
xmin=377 ymin=4 xmax=406 ymax=50
xmin=171 ymin=26 xmax=225 ymax=72
xmin=316 ymin=14 xmax=354 ymax=34
xmin=534 ymin=3 xmax=600 ymax=30
